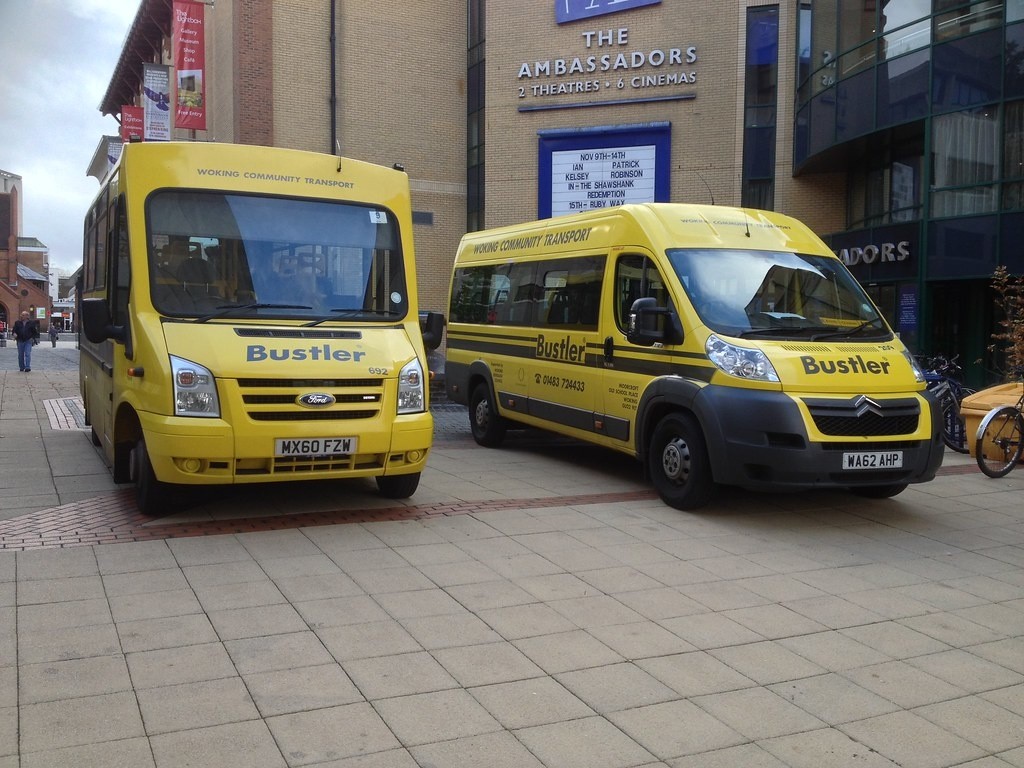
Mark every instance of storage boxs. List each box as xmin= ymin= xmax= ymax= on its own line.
xmin=959 ymin=382 xmax=1024 ymax=462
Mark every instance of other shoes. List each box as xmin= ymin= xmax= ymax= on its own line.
xmin=20 ymin=367 xmax=23 ymax=371
xmin=25 ymin=368 xmax=31 ymax=372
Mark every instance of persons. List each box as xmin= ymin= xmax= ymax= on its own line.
xmin=667 ymin=281 xmax=748 ymax=338
xmin=49 ymin=326 xmax=58 ymax=347
xmin=168 ymin=270 xmax=234 ymax=313
xmin=12 ymin=311 xmax=37 ymax=372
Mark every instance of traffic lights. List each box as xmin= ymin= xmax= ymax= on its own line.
xmin=30 ymin=305 xmax=35 ymax=319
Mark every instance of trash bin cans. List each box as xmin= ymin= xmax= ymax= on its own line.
xmin=960 ymin=382 xmax=1024 ymax=463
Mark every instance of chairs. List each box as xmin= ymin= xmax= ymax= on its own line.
xmin=457 ymin=277 xmax=658 ymax=331
xmin=148 ymin=241 xmax=324 ymax=315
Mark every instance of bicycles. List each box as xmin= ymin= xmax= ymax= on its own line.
xmin=913 ymin=353 xmax=978 ymax=454
xmin=975 ymin=392 xmax=1023 ymax=478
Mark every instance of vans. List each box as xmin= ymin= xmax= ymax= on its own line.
xmin=75 ymin=142 xmax=445 ymax=519
xmin=445 ymin=202 xmax=943 ymax=513
